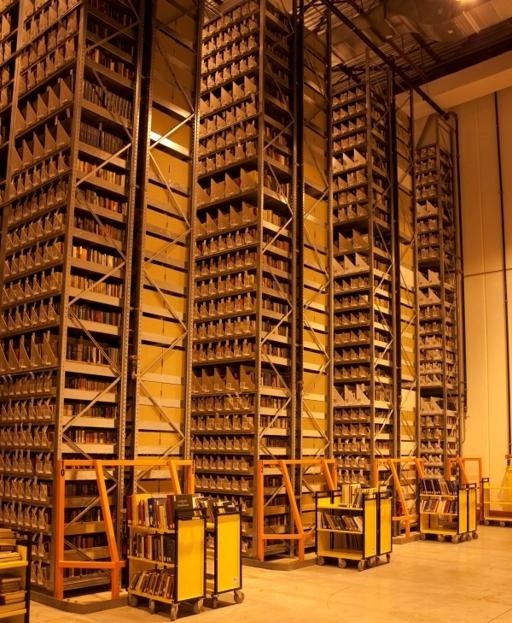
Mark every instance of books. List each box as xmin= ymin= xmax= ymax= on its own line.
xmin=414 ymin=146 xmax=460 ymax=515
xmin=0 ymin=0 xmax=138 ymax=603
xmin=132 ymin=493 xmax=238 ymax=601
xmin=322 ymin=483 xmax=389 ymax=550
xmin=191 ymin=1 xmax=290 ymax=551
xmin=332 ymin=86 xmax=394 ymax=485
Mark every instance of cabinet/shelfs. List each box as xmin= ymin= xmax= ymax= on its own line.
xmin=417 ymin=476 xmax=512 ymax=544
xmin=125 ymin=504 xmax=244 ymax=621
xmin=0 ymin=538 xmax=32 ymax=623
